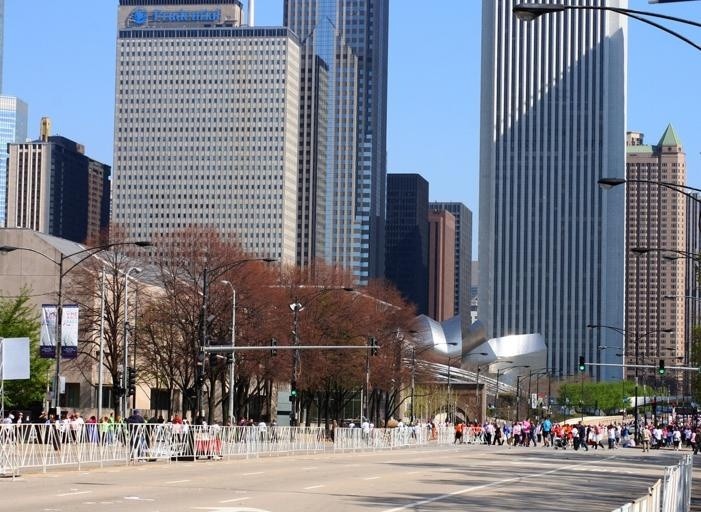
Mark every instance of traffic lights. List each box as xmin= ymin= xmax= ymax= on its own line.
xmin=270 ymin=337 xmax=277 ymax=358
xmin=290 ymin=381 xmax=297 ymax=395
xmin=130 ymin=368 xmax=136 ymax=395
xmin=580 ymin=356 xmax=584 ymax=370
xmin=225 ymin=342 xmax=234 ymax=364
xmin=208 ymin=339 xmax=218 ymax=365
xmin=375 ymin=341 xmax=379 ymax=356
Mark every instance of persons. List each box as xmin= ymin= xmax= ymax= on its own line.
xmin=379 ymin=415 xmax=438 ymax=446
xmin=454 ymin=416 xmax=551 ymax=448
xmin=550 ymin=420 xmax=635 ymax=451
xmin=339 ymin=419 xmax=355 ymax=439
xmin=363 ymin=419 xmax=374 ymax=440
xmin=0 ymin=405 xmax=280 ymax=463
xmin=331 ymin=419 xmax=340 ymax=444
xmin=638 ymin=414 xmax=700 ymax=455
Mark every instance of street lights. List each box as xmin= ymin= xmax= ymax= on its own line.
xmin=95 ymin=264 xmax=108 ymax=424
xmin=195 ymin=256 xmax=277 ymax=418
xmin=663 ymin=252 xmax=699 ymax=263
xmin=219 ymin=279 xmax=236 ymax=427
xmin=599 ymin=176 xmax=701 ymax=206
xmin=513 ymin=3 xmax=701 ymax=56
xmin=407 ymin=340 xmax=575 ymax=426
xmin=0 ymin=242 xmax=155 ymax=420
xmin=587 ymin=322 xmax=685 ymax=447
xmin=365 ymin=329 xmax=419 ymax=424
xmin=660 ymin=293 xmax=701 ymax=306
xmin=288 ymin=286 xmax=354 ymax=428
xmin=630 ymin=247 xmax=700 ymax=265
xmin=124 ymin=265 xmax=142 ymax=419
